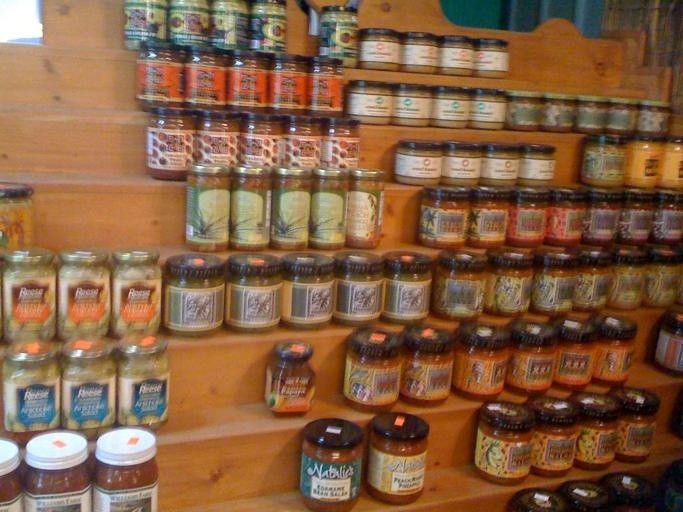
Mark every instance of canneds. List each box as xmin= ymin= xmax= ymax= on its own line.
xmin=0 ymin=183 xmax=37 ymax=254
xmin=416 ymin=192 xmax=683 ymax=251
xmin=352 ymin=79 xmax=683 ymax=191
xmin=474 ymin=389 xmax=664 ymax=511
xmin=257 ymin=342 xmax=318 ymax=416
xmin=343 ymin=317 xmax=637 ymax=412
xmin=369 ymin=414 xmax=433 ymax=505
xmin=123 ymin=2 xmax=515 ymax=79
xmin=162 ymin=251 xmax=682 ymax=338
xmin=294 ymin=413 xmax=360 ymax=511
xmin=652 ymin=314 xmax=682 ymax=376
xmin=137 ymin=44 xmax=386 ymax=248
xmin=1 ymin=250 xmax=170 ymax=511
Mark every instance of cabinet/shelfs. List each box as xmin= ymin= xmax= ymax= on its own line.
xmin=1 ymin=1 xmax=681 ymax=512
